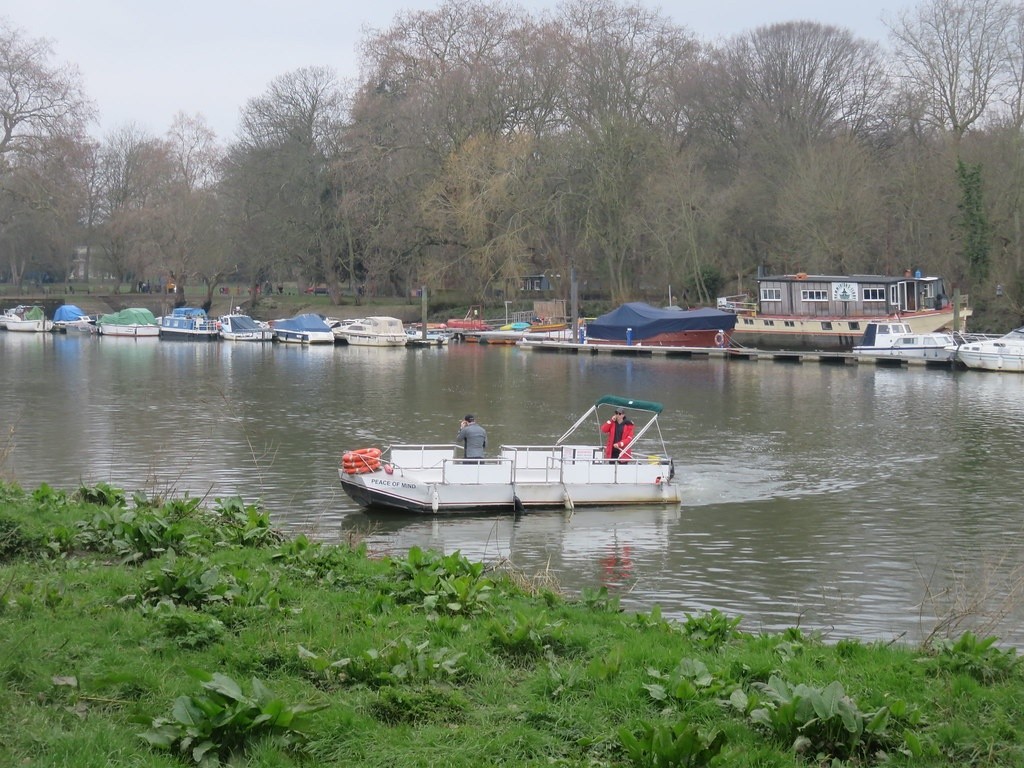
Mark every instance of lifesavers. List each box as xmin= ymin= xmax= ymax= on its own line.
xmin=343 ymin=448 xmax=381 ymax=462
xmin=714 ymin=333 xmax=724 ymax=345
xmin=344 ymin=459 xmax=379 ymax=467
xmin=344 ymin=463 xmax=379 ymax=475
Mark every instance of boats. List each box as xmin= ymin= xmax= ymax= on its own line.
xmin=1 ymin=300 xmax=579 ymax=348
xmin=944 ymin=325 xmax=1024 ymax=374
xmin=584 ymin=281 xmax=738 ymax=349
xmin=852 ymin=319 xmax=964 ymax=364
xmin=714 ymin=260 xmax=974 ymax=351
xmin=338 ymin=392 xmax=682 ymax=517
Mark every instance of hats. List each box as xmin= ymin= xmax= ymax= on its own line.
xmin=465 ymin=415 xmax=474 ymax=422
xmin=615 ymin=407 xmax=625 ymax=414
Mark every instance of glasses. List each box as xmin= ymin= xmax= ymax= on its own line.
xmin=616 ymin=413 xmax=621 ymax=415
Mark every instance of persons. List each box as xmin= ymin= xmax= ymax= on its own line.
xmin=138 ymin=278 xmax=177 ymax=293
xmin=248 ymin=279 xmax=284 ymax=294
xmin=600 ymin=407 xmax=636 ymax=465
xmin=456 ymin=414 xmax=487 ymax=464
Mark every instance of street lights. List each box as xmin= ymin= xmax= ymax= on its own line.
xmin=504 ymin=300 xmax=514 ymax=327
